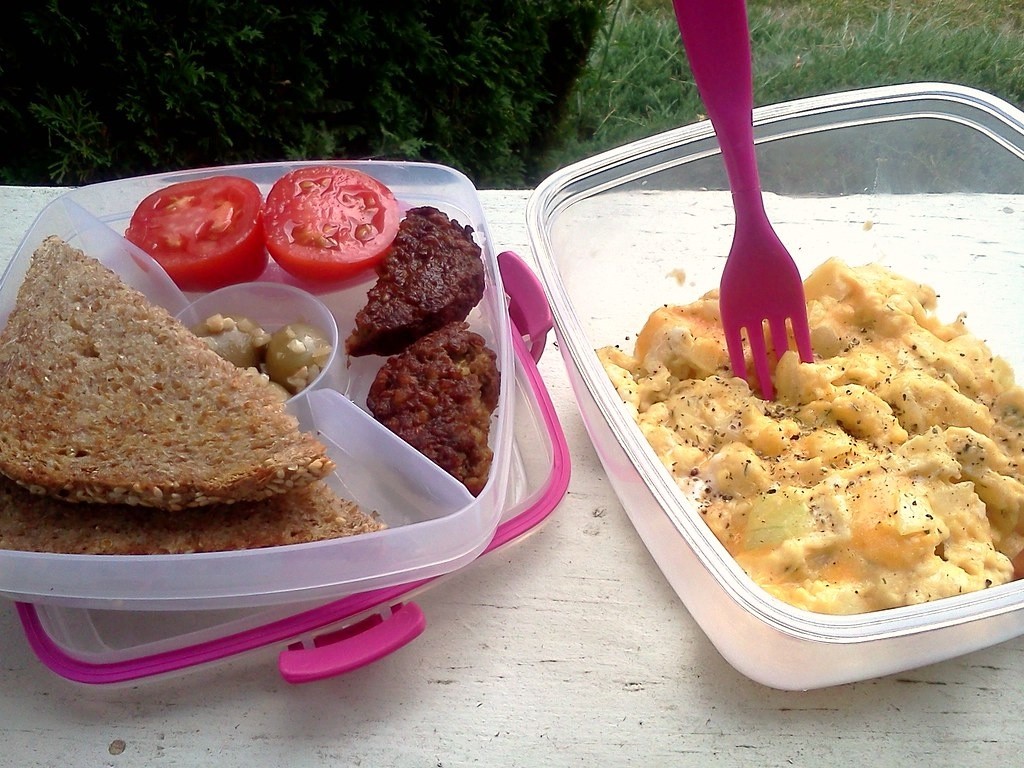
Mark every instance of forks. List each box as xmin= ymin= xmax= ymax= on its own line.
xmin=671 ymin=0 xmax=818 ymax=402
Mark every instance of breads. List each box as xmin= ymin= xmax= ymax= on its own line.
xmin=0 ymin=471 xmax=390 ymax=554
xmin=0 ymin=236 xmax=335 ymax=507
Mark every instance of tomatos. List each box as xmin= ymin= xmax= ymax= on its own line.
xmin=261 ymin=165 xmax=402 ymax=283
xmin=124 ymin=176 xmax=268 ymax=291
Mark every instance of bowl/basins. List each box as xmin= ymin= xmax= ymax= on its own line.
xmin=525 ymin=78 xmax=1024 ymax=690
xmin=0 ymin=157 xmax=509 ymax=610
